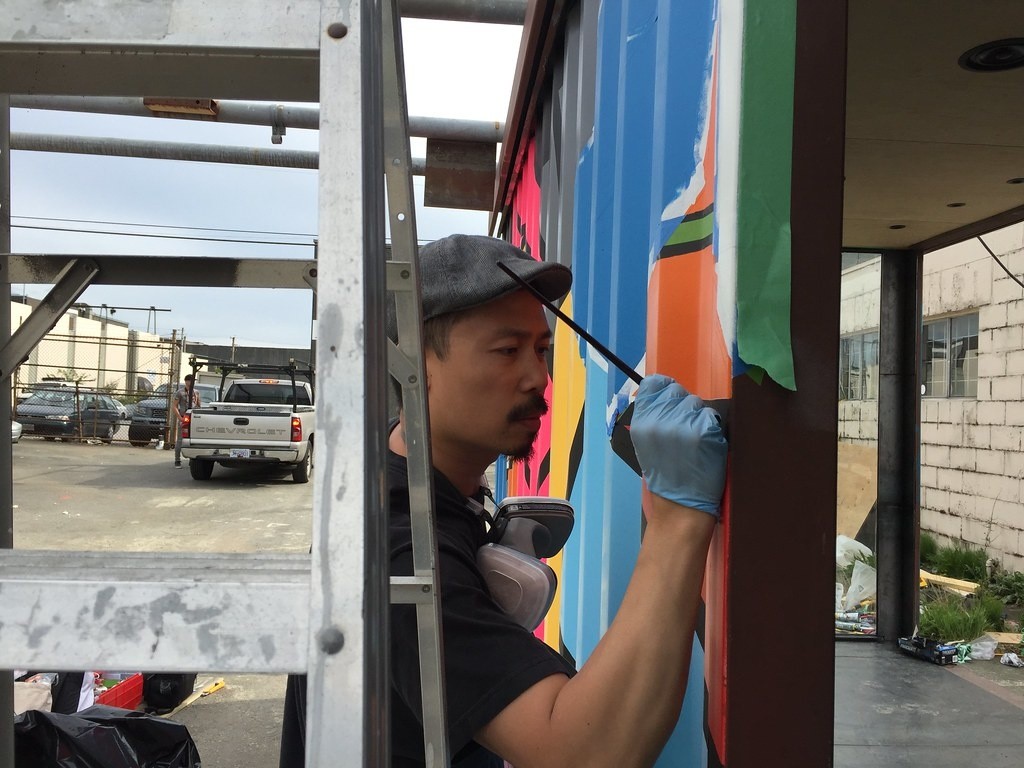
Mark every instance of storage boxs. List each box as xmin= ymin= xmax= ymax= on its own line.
xmin=94 ymin=671 xmax=144 ymax=711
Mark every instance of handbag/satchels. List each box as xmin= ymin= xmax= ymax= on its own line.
xmin=143 ymin=673 xmax=197 ymax=714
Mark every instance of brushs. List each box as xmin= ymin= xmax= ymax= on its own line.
xmin=497 ymin=259 xmax=643 ymax=387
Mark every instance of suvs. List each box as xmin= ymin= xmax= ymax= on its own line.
xmin=130 ymin=380 xmax=227 ymax=452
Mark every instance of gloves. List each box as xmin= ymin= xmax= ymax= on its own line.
xmin=630 ymin=373 xmax=725 ymax=518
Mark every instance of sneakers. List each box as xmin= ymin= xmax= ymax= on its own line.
xmin=175 ymin=461 xmax=181 ymax=468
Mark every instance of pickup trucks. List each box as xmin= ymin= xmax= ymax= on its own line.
xmin=181 ymin=379 xmax=314 ymax=483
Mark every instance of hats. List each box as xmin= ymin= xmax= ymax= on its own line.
xmin=389 ymin=234 xmax=573 ymax=343
xmin=185 ymin=374 xmax=197 ymax=380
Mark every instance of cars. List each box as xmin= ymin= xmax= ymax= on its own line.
xmin=15 ymin=390 xmax=121 ymax=444
xmin=11 ymin=420 xmax=23 ymax=443
xmin=110 ymin=398 xmax=128 ymax=420
xmin=16 ymin=381 xmax=76 ymax=405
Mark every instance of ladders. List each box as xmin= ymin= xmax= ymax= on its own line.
xmin=2 ymin=0 xmax=455 ymax=768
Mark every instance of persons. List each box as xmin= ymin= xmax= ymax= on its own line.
xmin=279 ymin=234 xmax=725 ymax=767
xmin=173 ymin=374 xmax=201 ymax=469
